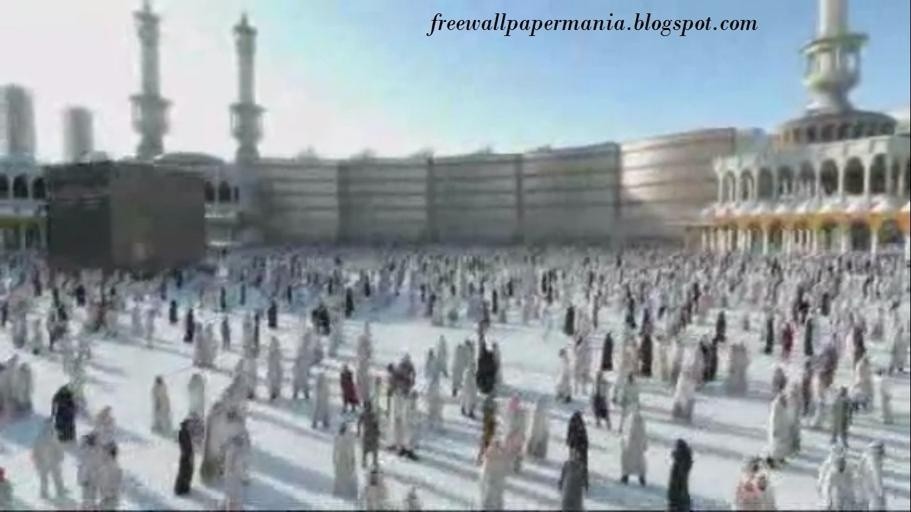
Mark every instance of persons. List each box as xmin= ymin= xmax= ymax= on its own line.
xmin=0 ymin=241 xmax=911 ymax=510
